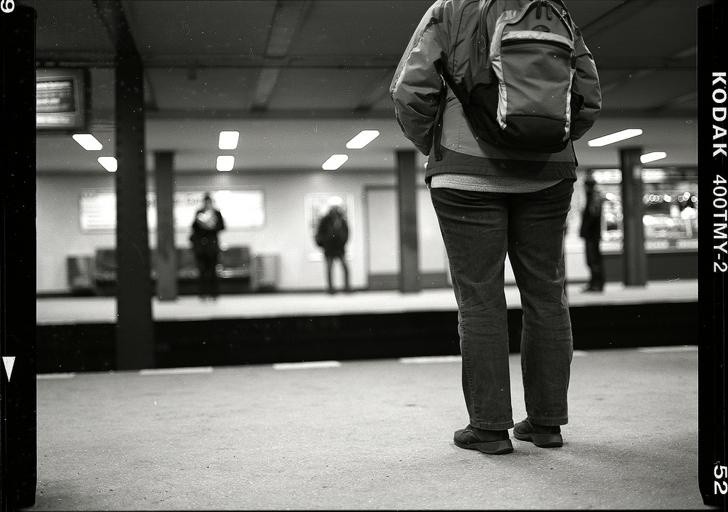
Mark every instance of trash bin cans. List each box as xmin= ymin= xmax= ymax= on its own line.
xmin=68 ymin=256 xmax=94 ymax=294
xmin=254 ymin=254 xmax=279 ymax=292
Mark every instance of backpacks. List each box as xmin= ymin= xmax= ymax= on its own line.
xmin=437 ymin=0 xmax=577 ymax=153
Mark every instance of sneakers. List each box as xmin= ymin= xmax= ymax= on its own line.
xmin=200 ymin=284 xmax=352 ymax=300
xmin=513 ymin=418 xmax=564 ymax=448
xmin=454 ymin=424 xmax=515 ymax=455
xmin=581 ymin=282 xmax=606 ymax=293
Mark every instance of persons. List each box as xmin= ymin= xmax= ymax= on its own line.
xmin=579 ymin=180 xmax=604 ymax=291
xmin=190 ymin=196 xmax=223 ymax=297
xmin=390 ymin=0 xmax=602 ymax=454
xmin=316 ymin=207 xmax=348 ymax=293
